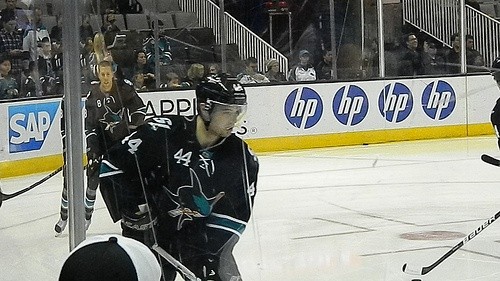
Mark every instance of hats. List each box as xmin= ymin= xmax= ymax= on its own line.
xmin=266 ymin=59 xmax=279 ymax=68
xmin=300 ymin=50 xmax=310 ymax=57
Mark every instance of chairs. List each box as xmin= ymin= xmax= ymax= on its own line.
xmin=29 ymin=0 xmax=246 ymax=81
xmin=476 ymin=0 xmax=500 ymax=19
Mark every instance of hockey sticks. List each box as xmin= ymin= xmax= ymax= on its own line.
xmin=1 ymin=165 xmax=64 ymax=201
xmin=84 ymin=128 xmax=130 ymax=169
xmin=148 ymin=243 xmax=203 ymax=281
xmin=402 ymin=211 xmax=499 ymax=275
xmin=481 ymin=154 xmax=499 ymax=167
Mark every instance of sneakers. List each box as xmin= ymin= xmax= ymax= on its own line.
xmin=55 ymin=219 xmax=68 ymax=233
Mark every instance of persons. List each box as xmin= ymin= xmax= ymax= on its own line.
xmin=1 ymin=95 xmax=68 ymax=233
xmin=287 ymin=49 xmax=316 ymax=81
xmin=80 ymin=12 xmax=231 ymax=92
xmin=264 ymin=58 xmax=286 ymax=82
xmin=317 ymin=33 xmax=487 ymax=81
xmin=488 ymin=58 xmax=500 ymax=151
xmin=237 ymin=57 xmax=269 ymax=84
xmin=99 ymin=72 xmax=259 ymax=281
xmin=82 ymin=61 xmax=147 ymax=232
xmin=0 ymin=7 xmax=64 ymax=100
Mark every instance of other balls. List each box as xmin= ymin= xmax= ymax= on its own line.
xmin=411 ymin=279 xmax=422 ymax=281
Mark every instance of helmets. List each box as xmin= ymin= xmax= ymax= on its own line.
xmin=196 ymin=73 xmax=248 ymax=122
xmin=490 ymin=57 xmax=500 ymax=76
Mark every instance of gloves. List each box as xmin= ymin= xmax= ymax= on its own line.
xmin=197 ymin=257 xmax=223 ymax=281
